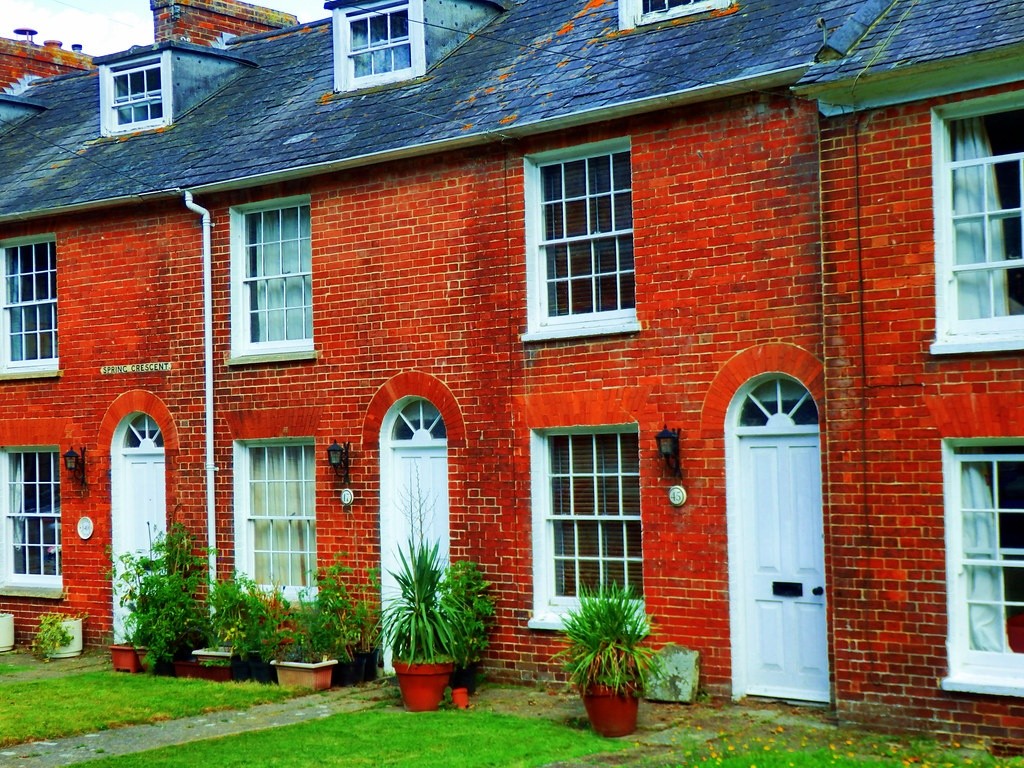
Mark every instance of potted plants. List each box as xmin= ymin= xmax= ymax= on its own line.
xmin=0 ymin=613 xmax=15 ymax=651
xmin=109 ymin=508 xmax=495 ymax=712
xmin=32 ymin=612 xmax=87 ymax=664
xmin=554 ymin=578 xmax=666 ymax=739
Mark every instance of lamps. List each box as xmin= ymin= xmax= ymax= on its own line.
xmin=64 ymin=445 xmax=91 ymax=487
xmin=327 ymin=439 xmax=351 ymax=483
xmin=656 ymin=424 xmax=682 ymax=476
